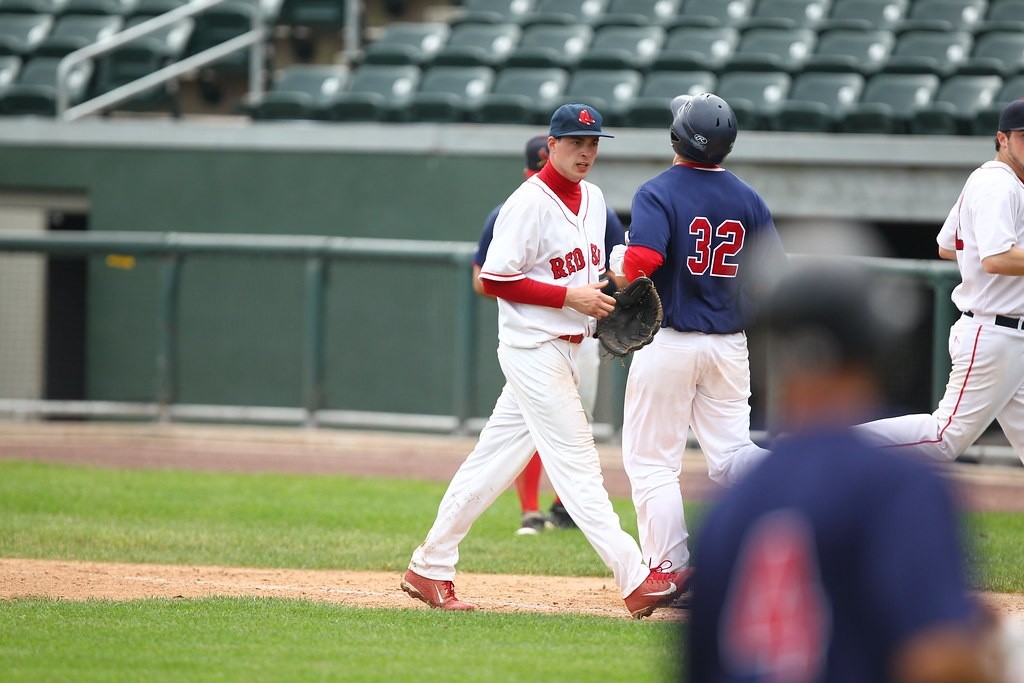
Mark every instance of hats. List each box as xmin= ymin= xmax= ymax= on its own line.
xmin=997 ymin=100 xmax=1024 ymax=131
xmin=550 ymin=102 xmax=614 ymax=138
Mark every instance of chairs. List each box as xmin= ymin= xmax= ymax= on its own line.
xmin=0 ymin=0 xmax=1024 ymax=139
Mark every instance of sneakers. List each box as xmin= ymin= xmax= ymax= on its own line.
xmin=400 ymin=569 xmax=475 ymax=611
xmin=623 ymin=560 xmax=696 ymax=619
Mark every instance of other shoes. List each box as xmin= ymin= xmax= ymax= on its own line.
xmin=551 ymin=506 xmax=575 ymax=528
xmin=518 ymin=511 xmax=545 ymax=535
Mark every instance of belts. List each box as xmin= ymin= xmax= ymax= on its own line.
xmin=964 ymin=308 xmax=1024 ymax=331
xmin=559 ymin=334 xmax=584 ymax=344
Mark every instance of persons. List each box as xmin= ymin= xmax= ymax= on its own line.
xmin=613 ymin=93 xmax=788 ymax=580
xmin=681 ymin=277 xmax=1013 ymax=683
xmin=843 ymin=104 xmax=1023 ymax=468
xmin=400 ymin=104 xmax=696 ymax=617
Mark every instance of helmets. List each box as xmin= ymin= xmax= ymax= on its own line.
xmin=668 ymin=93 xmax=738 ymax=165
xmin=526 ymin=136 xmax=549 ymax=170
